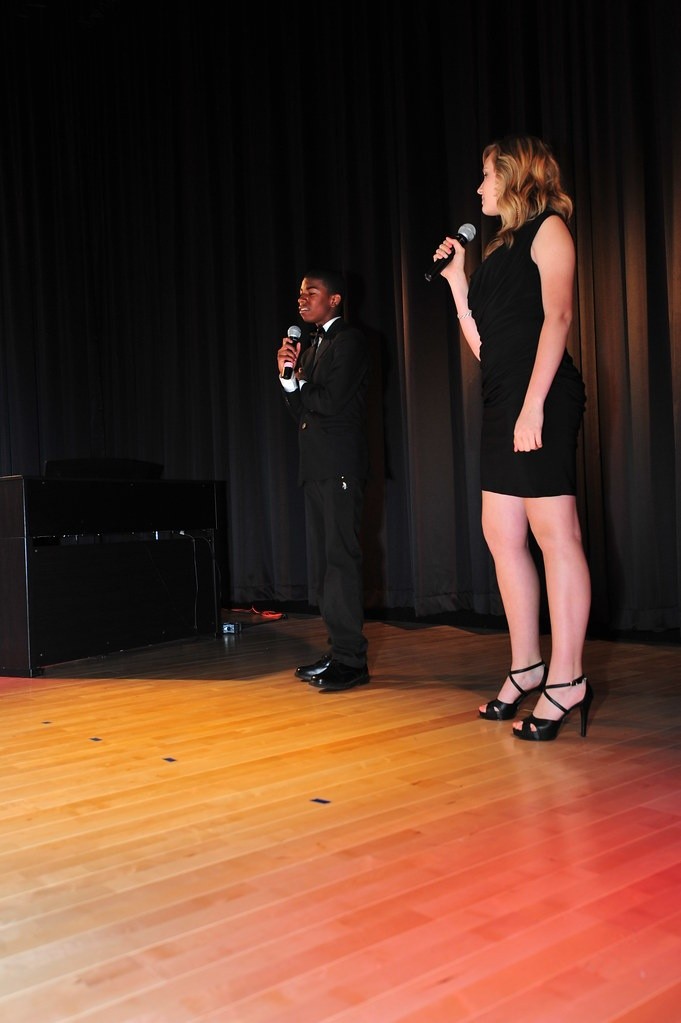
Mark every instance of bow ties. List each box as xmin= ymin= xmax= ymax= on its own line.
xmin=308 ymin=325 xmax=327 ymax=347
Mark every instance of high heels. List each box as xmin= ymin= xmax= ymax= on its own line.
xmin=512 ymin=674 xmax=593 ymax=741
xmin=478 ymin=659 xmax=548 ymax=721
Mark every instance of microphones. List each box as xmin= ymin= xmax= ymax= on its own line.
xmin=425 ymin=223 xmax=476 ymax=281
xmin=281 ymin=326 xmax=301 ymax=380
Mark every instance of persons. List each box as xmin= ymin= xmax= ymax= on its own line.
xmin=433 ymin=135 xmax=594 ymax=742
xmin=277 ymin=267 xmax=375 ymax=690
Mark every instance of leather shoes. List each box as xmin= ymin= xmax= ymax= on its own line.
xmin=308 ymin=657 xmax=370 ymax=690
xmin=294 ymin=652 xmax=338 ymax=680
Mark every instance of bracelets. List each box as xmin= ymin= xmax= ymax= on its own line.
xmin=458 ymin=313 xmax=471 ymax=318
